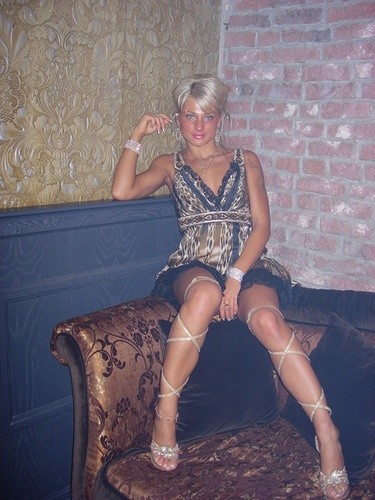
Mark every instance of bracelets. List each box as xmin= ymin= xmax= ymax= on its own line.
xmin=228 ymin=267 xmax=243 ymax=282
xmin=124 ymin=139 xmax=140 ymax=154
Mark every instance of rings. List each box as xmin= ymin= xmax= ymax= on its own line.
xmin=224 ymin=304 xmax=230 ymax=307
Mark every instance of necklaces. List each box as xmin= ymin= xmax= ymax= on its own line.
xmin=190 ymin=144 xmax=218 ymax=173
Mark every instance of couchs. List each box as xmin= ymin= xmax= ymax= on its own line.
xmin=52 ymin=285 xmax=375 ymax=500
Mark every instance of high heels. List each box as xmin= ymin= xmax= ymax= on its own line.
xmin=151 ymin=408 xmax=183 ymax=471
xmin=316 ymin=435 xmax=350 ymax=500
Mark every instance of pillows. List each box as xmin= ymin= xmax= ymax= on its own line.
xmin=283 ymin=313 xmax=375 ymax=481
xmin=157 ymin=317 xmax=280 ymax=444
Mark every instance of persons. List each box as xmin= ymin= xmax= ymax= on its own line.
xmin=111 ymin=73 xmax=351 ymax=500
